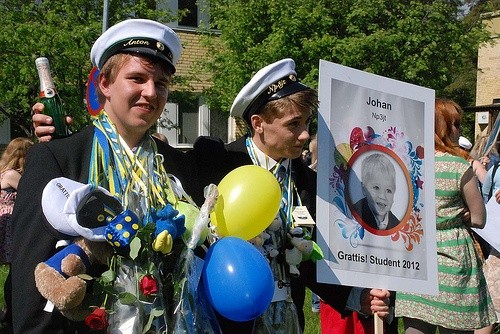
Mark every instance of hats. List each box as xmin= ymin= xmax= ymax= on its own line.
xmin=230 ymin=58 xmax=310 ymax=127
xmin=90 ymin=19 xmax=182 ymax=75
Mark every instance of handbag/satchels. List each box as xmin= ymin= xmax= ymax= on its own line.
xmin=474 ymin=233 xmax=492 ymax=260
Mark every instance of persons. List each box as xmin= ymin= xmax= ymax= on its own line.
xmin=33 ymin=57 xmax=316 ymax=334
xmin=0 ymin=139 xmax=33 ymax=329
xmin=14 ymin=19 xmax=205 ymax=334
xmin=307 ymin=99 xmax=500 ymax=334
xmin=351 ymin=153 xmax=402 ymax=230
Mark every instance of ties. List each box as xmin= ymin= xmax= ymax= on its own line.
xmin=272 ymin=164 xmax=286 ymax=192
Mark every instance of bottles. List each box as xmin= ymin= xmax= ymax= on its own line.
xmin=34 ymin=58 xmax=68 ymax=140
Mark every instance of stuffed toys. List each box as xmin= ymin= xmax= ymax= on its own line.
xmin=247 ymin=237 xmax=269 ymax=264
xmin=263 ymin=218 xmax=281 ymax=257
xmin=33 ymin=205 xmax=186 ymax=320
xmin=283 ymin=228 xmax=313 ymax=277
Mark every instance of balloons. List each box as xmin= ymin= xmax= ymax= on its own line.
xmin=210 ymin=164 xmax=281 ymax=241
xmin=200 ymin=238 xmax=274 ymax=320
xmin=174 ymin=200 xmax=208 ymax=247
